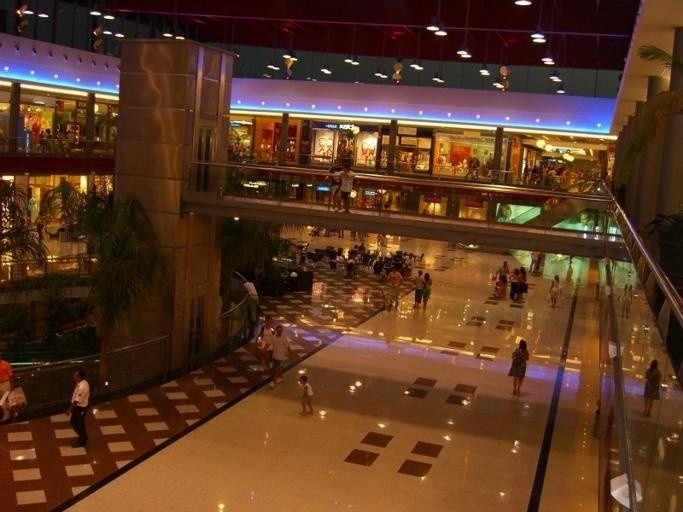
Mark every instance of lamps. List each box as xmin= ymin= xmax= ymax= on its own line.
xmin=17 ymin=1 xmax=567 ymax=95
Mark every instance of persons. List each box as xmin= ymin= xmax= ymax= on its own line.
xmin=332 ymin=161 xmax=356 ymax=215
xmin=625 ymin=284 xmax=633 ymax=316
xmin=328 ymin=159 xmax=342 ymax=208
xmin=508 ymin=340 xmax=529 ymax=396
xmin=388 ymin=191 xmax=393 ymax=205
xmin=255 ymin=316 xmax=278 ymax=367
xmin=245 ymin=145 xmax=251 ymax=158
xmin=491 ymin=245 xmax=563 ymax=308
xmin=65 ymin=369 xmax=91 ymax=448
xmin=36 ymin=128 xmax=75 ymax=154
xmin=36 ymin=209 xmax=74 ymax=243
xmin=0 ymin=356 xmax=18 ymax=423
xmin=299 ymin=375 xmax=314 ymax=415
xmin=457 ymin=158 xmax=612 ymax=195
xmin=241 ymin=277 xmax=262 ymax=324
xmin=263 ymin=325 xmax=293 ymax=389
xmin=622 ymin=285 xmax=628 ymax=319
xmin=309 ymin=226 xmax=432 ymax=309
xmin=641 ymin=359 xmax=662 ymax=419
